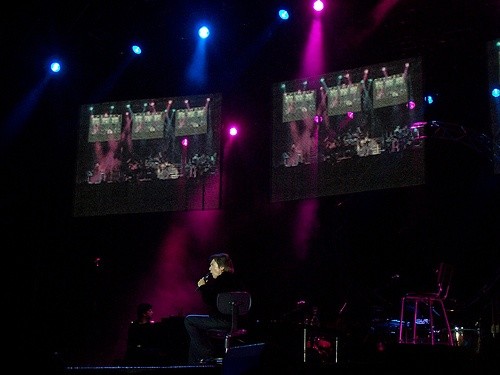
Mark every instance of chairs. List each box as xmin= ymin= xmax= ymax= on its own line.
xmin=398 ymin=262 xmax=453 ymax=347
xmin=200 ymin=292 xmax=251 ymax=365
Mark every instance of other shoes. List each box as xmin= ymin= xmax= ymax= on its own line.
xmin=199 ymin=357 xmax=222 ymax=365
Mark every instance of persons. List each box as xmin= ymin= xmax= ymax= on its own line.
xmin=184 ymin=254 xmax=246 ymax=366
xmin=161 ymin=151 xmax=169 ymax=163
xmin=87 ymin=168 xmax=102 ymax=184
xmin=405 ymin=125 xmax=410 ymax=139
xmin=357 ymin=132 xmax=372 ymax=151
xmin=320 ymin=129 xmax=341 ymax=157
xmin=124 ymin=158 xmax=145 ymax=184
xmin=128 ymin=303 xmax=155 ymax=324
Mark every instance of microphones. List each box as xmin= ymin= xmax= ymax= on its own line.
xmin=195 ymin=273 xmax=210 ymax=292
